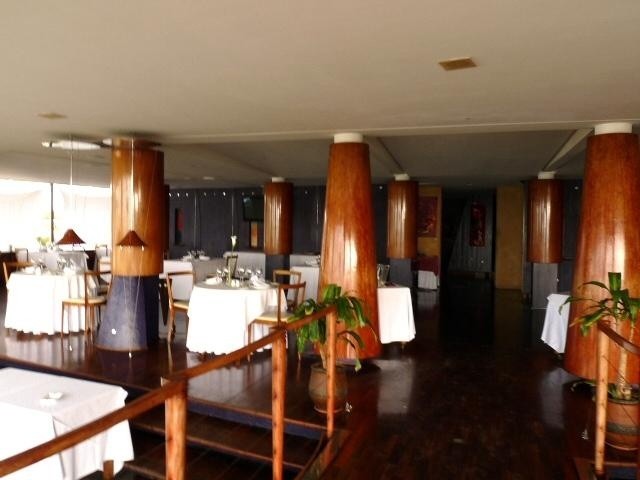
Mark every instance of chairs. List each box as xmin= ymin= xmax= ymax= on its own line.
xmin=2 ymin=244 xmax=115 ymax=347
xmin=246 ymin=281 xmax=306 ymax=363
xmin=164 ymin=247 xmax=320 ymax=277
xmin=165 ymin=270 xmax=196 ymax=344
xmin=272 ymin=267 xmax=302 ymax=310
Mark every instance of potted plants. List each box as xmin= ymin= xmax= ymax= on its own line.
xmin=555 ymin=272 xmax=640 ymax=454
xmin=290 ymin=281 xmax=378 ymax=415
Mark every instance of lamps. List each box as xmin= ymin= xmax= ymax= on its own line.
xmin=51 ymin=131 xmax=87 ymax=248
xmin=112 ymin=138 xmax=150 ymax=251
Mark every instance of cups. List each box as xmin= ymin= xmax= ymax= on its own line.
xmin=377 ymin=264 xmax=390 ymax=286
xmin=215 ymin=265 xmax=263 ymax=288
xmin=27 ymin=256 xmax=76 ymax=275
xmin=187 ymin=250 xmax=205 ymax=262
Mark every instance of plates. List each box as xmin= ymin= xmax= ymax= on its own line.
xmin=40 ymin=391 xmax=64 ymax=401
